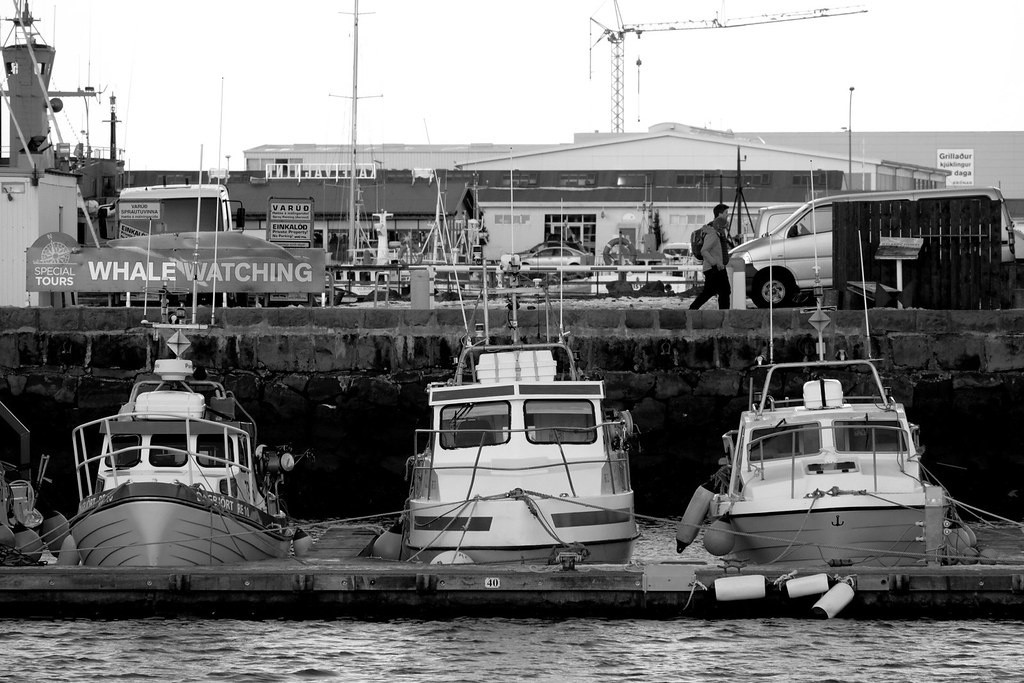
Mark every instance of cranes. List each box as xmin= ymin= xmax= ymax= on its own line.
xmin=589 ymin=0 xmax=868 ymax=134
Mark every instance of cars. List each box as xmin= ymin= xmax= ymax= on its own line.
xmin=506 ymin=239 xmax=593 ymax=267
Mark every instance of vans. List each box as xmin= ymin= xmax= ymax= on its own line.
xmin=727 ymin=189 xmax=1017 ymax=308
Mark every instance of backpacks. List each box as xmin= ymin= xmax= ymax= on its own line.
xmin=690 ymin=229 xmax=706 ymax=260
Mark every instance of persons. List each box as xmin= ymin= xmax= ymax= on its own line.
xmin=688 ymin=205 xmax=735 ymax=310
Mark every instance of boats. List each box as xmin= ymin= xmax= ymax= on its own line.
xmin=68 ymin=143 xmax=288 ymax=567
xmin=393 ymin=149 xmax=643 ymax=567
xmin=677 ymin=162 xmax=980 ymax=566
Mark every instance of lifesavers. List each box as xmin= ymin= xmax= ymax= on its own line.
xmin=603 ymin=238 xmax=637 ymax=272
